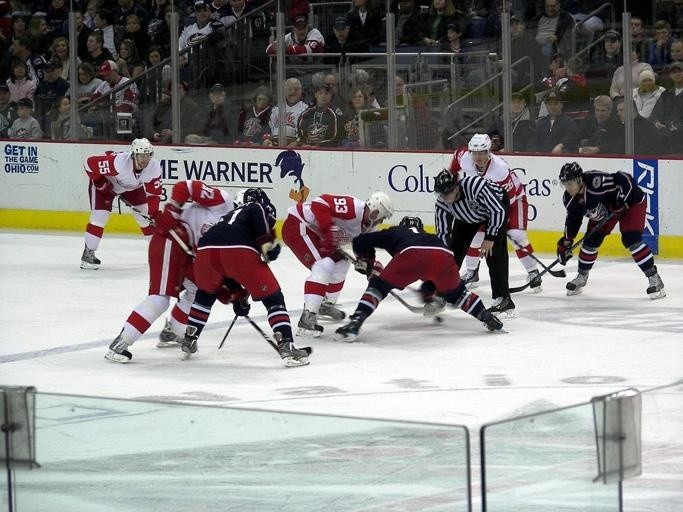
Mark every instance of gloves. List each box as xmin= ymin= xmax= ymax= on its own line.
xmin=95 ymin=177 xmax=113 ymax=193
xmin=153 ymin=204 xmax=183 ymax=236
xmin=233 ymin=299 xmax=250 ymax=317
xmin=261 ymin=242 xmax=280 ymax=260
xmin=354 ymin=257 xmax=372 ymax=275
xmin=319 ymin=226 xmax=343 ymax=257
xmin=557 ymin=237 xmax=573 ymax=266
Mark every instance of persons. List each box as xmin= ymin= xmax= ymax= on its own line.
xmin=180 ymin=187 xmax=312 ymax=359
xmin=433 ymin=168 xmax=516 ymax=314
xmin=2 ymin=0 xmax=682 ymax=156
xmin=557 ymin=162 xmax=665 ymax=295
xmin=449 ymin=132 xmax=542 ymax=289
xmin=80 ymin=137 xmax=163 ymax=265
xmin=335 ymin=216 xmax=504 ymax=336
xmin=109 ymin=179 xmax=248 ymax=360
xmin=281 ymin=191 xmax=395 ymax=334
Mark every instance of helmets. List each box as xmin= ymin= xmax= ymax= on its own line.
xmin=468 ymin=133 xmax=491 ymax=153
xmin=130 ymin=138 xmax=154 ymax=153
xmin=366 ymin=192 xmax=393 ymax=221
xmin=399 ymin=216 xmax=423 ymax=229
xmin=233 ymin=188 xmax=270 ymax=208
xmin=559 ymin=162 xmax=583 ymax=182
xmin=434 ymin=169 xmax=457 ymax=195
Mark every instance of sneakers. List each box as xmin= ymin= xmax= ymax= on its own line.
xmin=159 ymin=318 xmax=197 ymax=353
xmin=647 ymin=273 xmax=664 ymax=293
xmin=486 ymin=296 xmax=515 ymax=331
xmin=81 ymin=243 xmax=101 ymax=264
xmin=109 ymin=327 xmax=132 ymax=360
xmin=460 ymin=261 xmax=480 ymax=284
xmin=298 ymin=299 xmax=361 ymax=335
xmin=528 ymin=269 xmax=542 ymax=288
xmin=278 ymin=341 xmax=311 ymax=361
xmin=566 ymin=273 xmax=588 ymax=290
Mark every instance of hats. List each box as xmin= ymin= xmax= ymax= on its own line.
xmin=334 ymin=16 xmax=349 ymax=27
xmin=97 ymin=60 xmax=119 ymax=75
xmin=0 ymin=84 xmax=33 ymax=106
xmin=669 ymin=62 xmax=683 ymax=72
xmin=315 ymin=83 xmax=332 ymax=92
xmin=512 ymin=91 xmax=564 ymax=101
xmin=293 ymin=16 xmax=309 ymax=27
xmin=605 ymin=31 xmax=620 ymax=38
xmin=210 ymin=83 xmax=223 ymax=91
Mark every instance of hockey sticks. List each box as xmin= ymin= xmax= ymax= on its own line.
xmin=508 ymin=234 xmax=578 ymax=278
xmin=244 ymin=313 xmax=312 ymax=359
xmin=507 ymin=210 xmax=616 ymax=293
xmin=309 ymin=224 xmax=446 ymax=313
